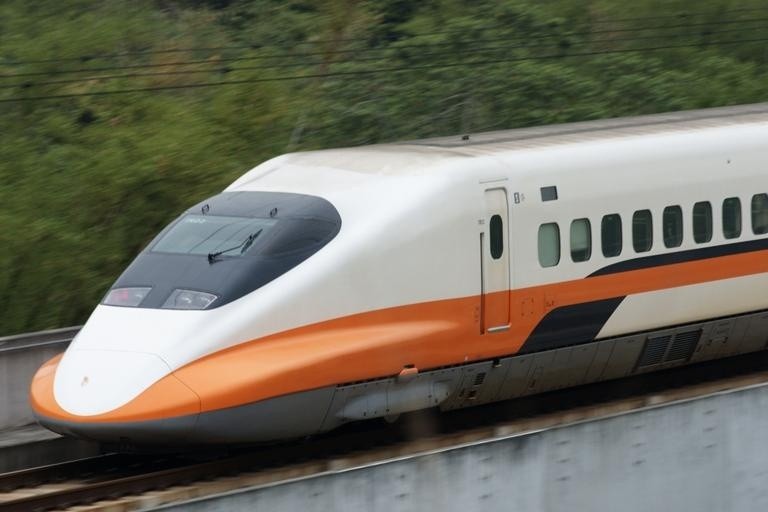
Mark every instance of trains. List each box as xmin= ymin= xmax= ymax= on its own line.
xmin=28 ymin=101 xmax=768 ymax=447
